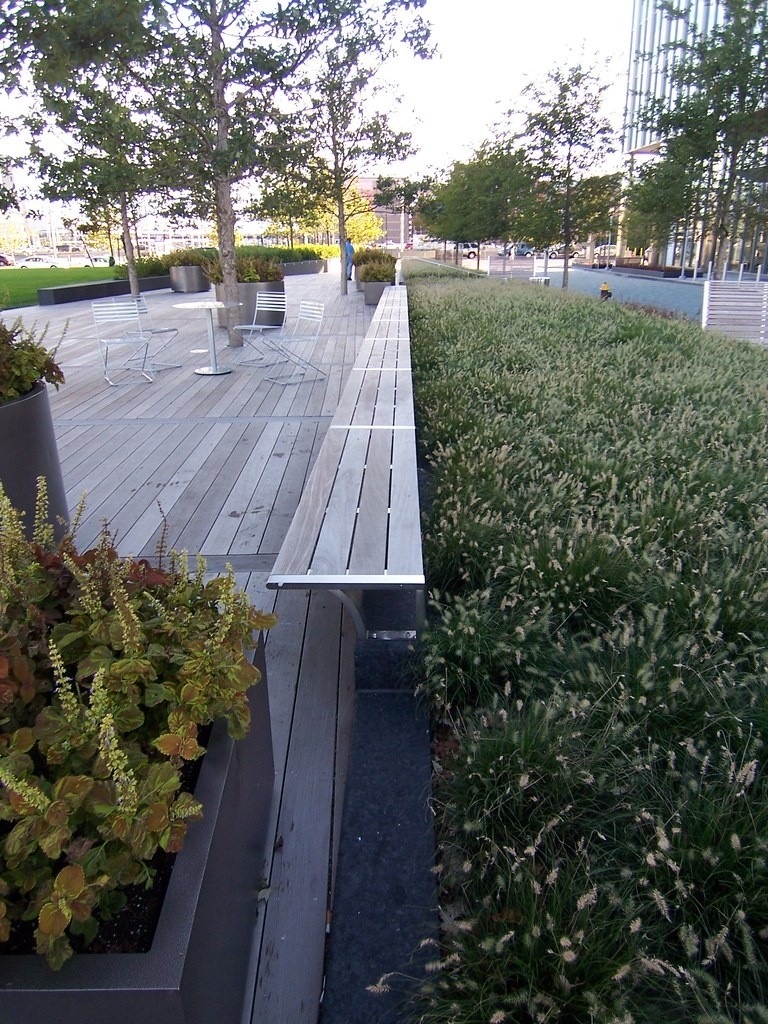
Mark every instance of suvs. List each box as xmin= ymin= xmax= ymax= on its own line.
xmin=56 ymin=245 xmax=80 ymax=251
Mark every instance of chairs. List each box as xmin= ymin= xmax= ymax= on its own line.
xmin=229 ymin=292 xmax=329 ymax=387
xmin=91 ymin=293 xmax=182 ymax=386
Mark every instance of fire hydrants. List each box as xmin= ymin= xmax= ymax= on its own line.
xmin=600 ymin=282 xmax=613 ymax=300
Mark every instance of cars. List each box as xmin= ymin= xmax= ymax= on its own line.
xmin=544 ymin=242 xmax=586 ymax=260
xmin=16 ymin=255 xmax=60 ymax=269
xmin=0 ymin=254 xmax=14 ymax=266
xmin=424 ymin=236 xmax=545 ymax=261
xmin=594 ymin=244 xmax=633 ymax=258
xmin=81 ymin=256 xmax=116 ymax=267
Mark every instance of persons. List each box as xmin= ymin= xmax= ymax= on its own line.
xmin=510 ymin=246 xmax=514 ymax=260
xmin=345 ymin=238 xmax=354 ymax=281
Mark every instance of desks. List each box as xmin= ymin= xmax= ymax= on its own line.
xmin=173 ymin=301 xmax=243 ymax=375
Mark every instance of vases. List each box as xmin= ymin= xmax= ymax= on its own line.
xmin=1 ymin=629 xmax=273 ymax=1024
xmin=0 ymin=381 xmax=70 ymax=551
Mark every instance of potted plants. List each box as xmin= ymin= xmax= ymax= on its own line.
xmin=351 ymin=246 xmax=397 ymax=305
xmin=167 ymin=244 xmax=286 ymax=328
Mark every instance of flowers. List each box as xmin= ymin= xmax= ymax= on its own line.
xmin=0 ymin=477 xmax=279 ymax=967
xmin=0 ymin=313 xmax=70 ymax=405
